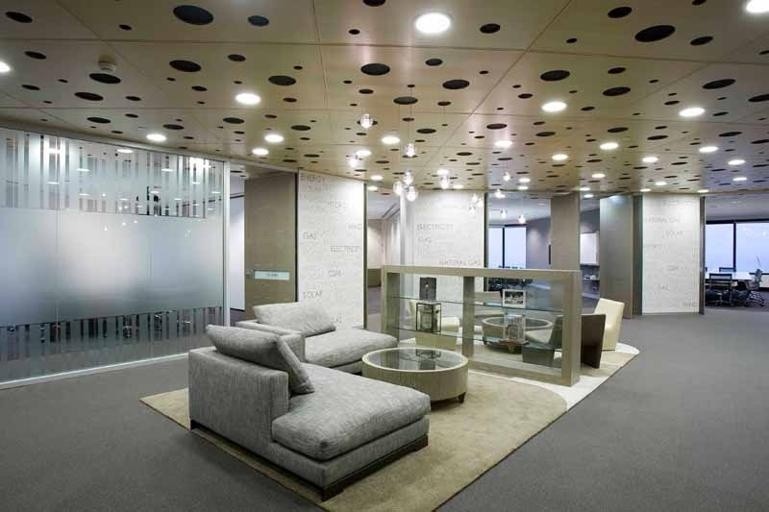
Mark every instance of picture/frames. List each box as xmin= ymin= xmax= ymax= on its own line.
xmin=502 ymin=288 xmax=527 ymax=308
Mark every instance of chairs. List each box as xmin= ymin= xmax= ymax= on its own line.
xmin=593 ymin=298 xmax=625 ymax=351
xmin=705 ymin=267 xmax=762 ymax=307
xmin=525 ymin=314 xmax=606 ymax=369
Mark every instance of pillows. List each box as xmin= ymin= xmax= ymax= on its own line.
xmin=205 ymin=324 xmax=316 ymax=395
xmin=251 ymin=301 xmax=336 ymax=338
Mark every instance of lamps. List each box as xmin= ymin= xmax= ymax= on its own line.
xmin=354 ymin=101 xmax=486 ymax=217
xmin=518 ymin=215 xmax=526 ymax=224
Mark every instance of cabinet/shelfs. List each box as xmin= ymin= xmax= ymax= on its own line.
xmin=415 ymin=301 xmax=442 ymax=333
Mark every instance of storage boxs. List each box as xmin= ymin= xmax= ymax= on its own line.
xmin=522 ymin=343 xmax=554 ymax=367
xmin=520 ymin=340 xmax=555 ymax=367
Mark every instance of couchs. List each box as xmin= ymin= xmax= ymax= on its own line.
xmin=235 ymin=318 xmax=397 ymax=373
xmin=188 ymin=334 xmax=432 ymax=502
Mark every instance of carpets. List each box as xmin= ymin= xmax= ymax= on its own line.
xmin=138 ymin=341 xmax=640 ymax=512
